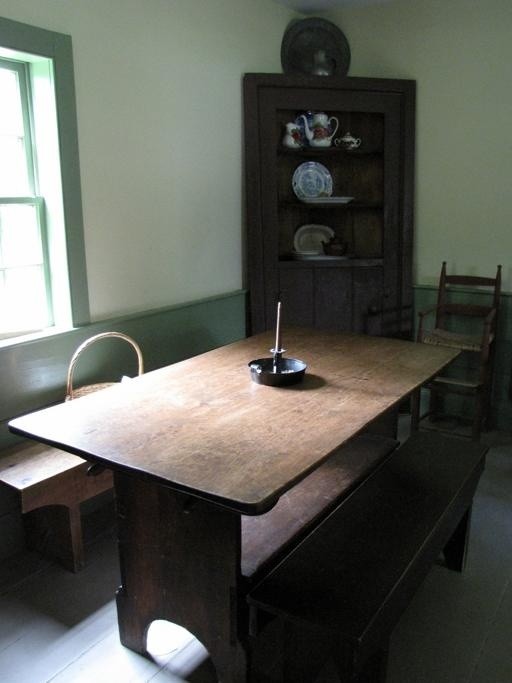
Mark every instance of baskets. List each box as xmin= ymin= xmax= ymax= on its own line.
xmin=65 ymin=331 xmax=144 ymax=402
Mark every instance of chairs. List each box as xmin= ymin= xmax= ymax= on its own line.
xmin=411 ymin=261 xmax=502 ymax=442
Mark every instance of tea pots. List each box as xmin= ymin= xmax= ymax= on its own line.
xmin=320 ymin=235 xmax=352 ymax=255
xmin=300 ymin=112 xmax=340 ymax=147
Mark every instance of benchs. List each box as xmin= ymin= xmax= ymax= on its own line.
xmin=241 ymin=428 xmax=490 ymax=683
xmin=1 ymin=437 xmax=114 ymax=575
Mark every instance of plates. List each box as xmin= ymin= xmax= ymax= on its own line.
xmin=294 ymin=111 xmax=334 ymax=147
xmin=297 ymin=196 xmax=355 ymax=204
xmin=291 ymin=222 xmax=346 ymax=262
xmin=292 ymin=161 xmax=334 ymax=198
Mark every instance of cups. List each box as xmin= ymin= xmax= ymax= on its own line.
xmin=284 ymin=122 xmax=304 ymax=149
xmin=312 ymin=48 xmax=338 ymax=77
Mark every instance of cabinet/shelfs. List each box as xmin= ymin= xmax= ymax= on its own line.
xmin=241 ymin=70 xmax=417 ymax=341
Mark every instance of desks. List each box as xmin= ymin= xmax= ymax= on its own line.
xmin=6 ymin=325 xmax=461 ymax=681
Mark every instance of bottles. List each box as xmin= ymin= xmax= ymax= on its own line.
xmin=334 ymin=131 xmax=362 ymax=151
